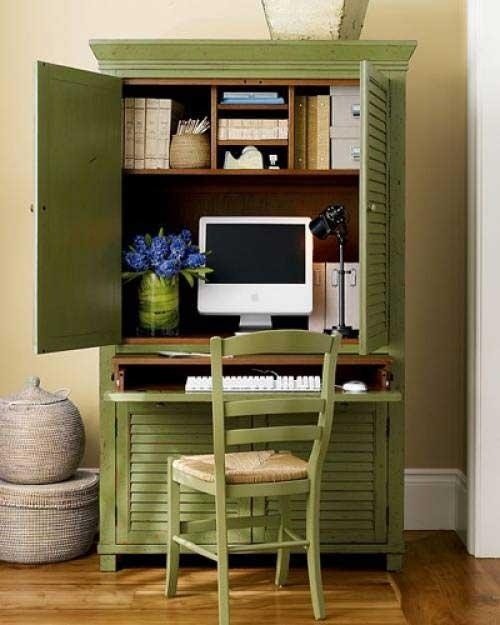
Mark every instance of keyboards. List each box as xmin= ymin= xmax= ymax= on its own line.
xmin=184 ymin=375 xmax=322 ymax=393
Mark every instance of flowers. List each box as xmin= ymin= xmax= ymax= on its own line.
xmin=122 ymin=227 xmax=215 ymax=288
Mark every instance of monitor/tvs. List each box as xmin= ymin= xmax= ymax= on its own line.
xmin=198 ymin=216 xmax=315 ymax=339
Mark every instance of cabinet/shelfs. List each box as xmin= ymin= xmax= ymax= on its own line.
xmin=33 ymin=39 xmax=418 ymax=572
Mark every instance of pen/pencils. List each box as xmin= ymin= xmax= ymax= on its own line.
xmin=176 ymin=115 xmax=211 ymax=135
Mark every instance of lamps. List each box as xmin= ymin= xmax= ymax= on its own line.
xmin=309 ymin=204 xmax=359 ymax=339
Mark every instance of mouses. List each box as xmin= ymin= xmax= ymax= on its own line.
xmin=341 ymin=379 xmax=369 ymax=392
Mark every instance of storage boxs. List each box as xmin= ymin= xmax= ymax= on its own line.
xmin=329 ymin=85 xmax=360 ymax=169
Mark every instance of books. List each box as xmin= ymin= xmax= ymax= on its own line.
xmin=223 ymin=91 xmax=278 ymax=100
xmin=293 ymin=95 xmax=306 ymax=172
xmin=220 ymin=97 xmax=285 ymax=105
xmin=316 ymin=94 xmax=332 ymax=171
xmin=120 ymin=96 xmax=185 ymax=171
xmin=304 ymin=94 xmax=319 ymax=172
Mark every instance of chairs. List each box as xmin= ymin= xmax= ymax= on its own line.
xmin=164 ymin=330 xmax=342 ymax=625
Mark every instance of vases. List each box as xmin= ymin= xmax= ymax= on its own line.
xmin=138 ymin=273 xmax=179 ymax=337
